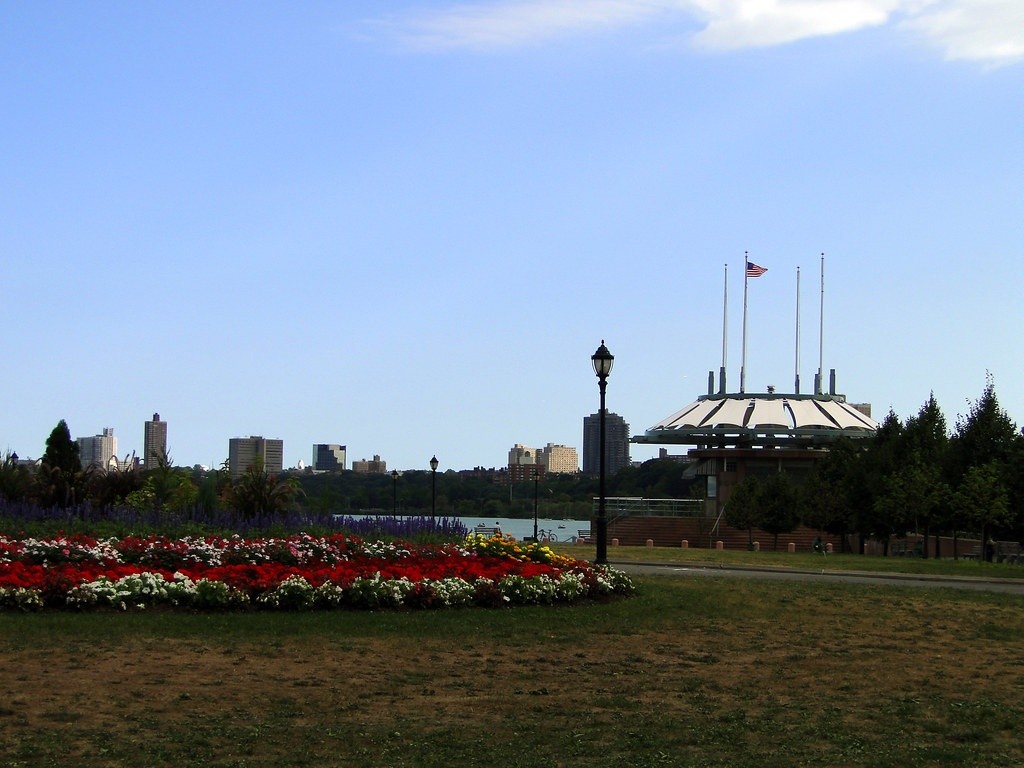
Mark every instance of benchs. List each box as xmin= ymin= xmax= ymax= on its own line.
xmin=962 ymin=546 xmax=980 ymax=560
xmin=996 ymin=541 xmax=1024 ymax=566
xmin=474 ymin=527 xmax=498 ymax=540
xmin=578 ymin=530 xmax=597 ymax=545
xmin=890 ymin=544 xmax=912 ymax=557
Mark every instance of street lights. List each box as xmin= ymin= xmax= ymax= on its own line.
xmin=390 ymin=469 xmax=399 ymax=520
xmin=429 ymin=454 xmax=440 ymax=533
xmin=589 ymin=340 xmax=616 ymax=566
xmin=531 ymin=470 xmax=541 ymax=539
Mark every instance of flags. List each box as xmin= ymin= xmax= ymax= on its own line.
xmin=746 ymin=261 xmax=768 ymax=277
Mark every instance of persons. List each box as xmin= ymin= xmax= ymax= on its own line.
xmin=494 ymin=521 xmax=502 ymax=538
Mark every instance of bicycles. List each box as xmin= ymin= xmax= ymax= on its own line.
xmin=536 ymin=529 xmax=559 ymax=543
